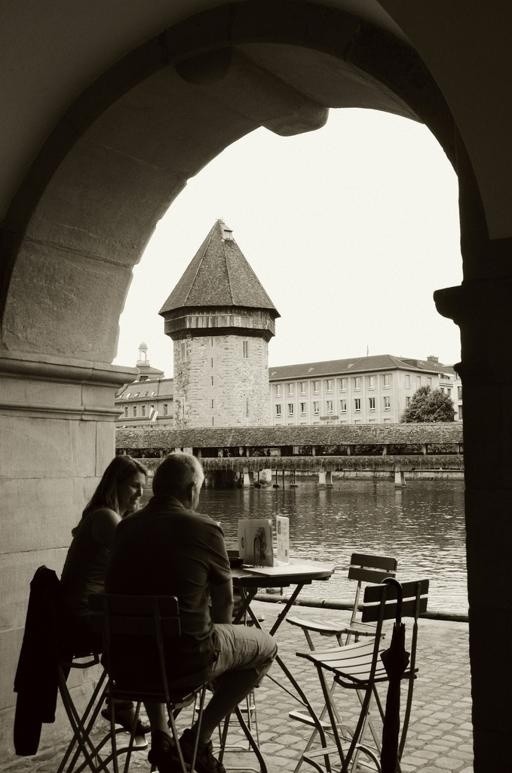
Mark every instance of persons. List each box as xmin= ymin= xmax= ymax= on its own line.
xmin=61 ymin=455 xmax=147 ymax=736
xmin=99 ymin=452 xmax=277 ymax=773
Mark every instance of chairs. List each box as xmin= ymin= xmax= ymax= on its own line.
xmin=34 ymin=566 xmax=265 ymax=766
xmin=285 ymin=553 xmax=429 ymax=772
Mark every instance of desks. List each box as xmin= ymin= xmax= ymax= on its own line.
xmin=213 ymin=561 xmax=339 ymax=773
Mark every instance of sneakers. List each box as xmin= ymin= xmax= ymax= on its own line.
xmin=102 ymin=702 xmax=152 ymax=733
xmin=173 ymin=728 xmax=225 ymax=772
xmin=148 ymin=730 xmax=179 ymax=773
